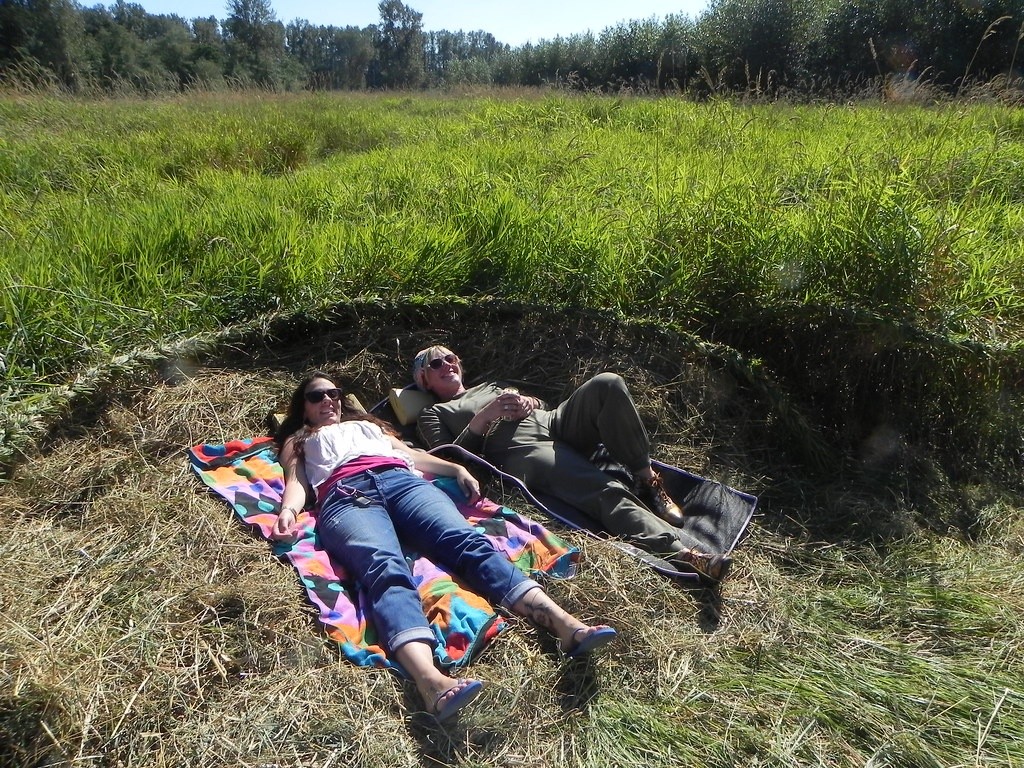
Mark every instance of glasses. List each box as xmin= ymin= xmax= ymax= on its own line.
xmin=427 ymin=354 xmax=458 ymax=369
xmin=305 ymin=388 xmax=343 ymax=404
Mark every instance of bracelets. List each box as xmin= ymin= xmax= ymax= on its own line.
xmin=278 ymin=506 xmax=298 ymax=522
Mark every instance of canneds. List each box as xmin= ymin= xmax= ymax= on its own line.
xmin=501 ymin=387 xmax=520 ymax=423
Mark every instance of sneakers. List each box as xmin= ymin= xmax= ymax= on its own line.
xmin=683 ymin=545 xmax=733 ymax=581
xmin=640 ymin=470 xmax=685 ymax=527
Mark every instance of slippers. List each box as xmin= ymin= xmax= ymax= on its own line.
xmin=569 ymin=628 xmax=617 ymax=656
xmin=432 ymin=681 xmax=482 ymax=721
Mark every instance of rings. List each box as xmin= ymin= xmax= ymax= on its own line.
xmin=505 ymin=404 xmax=508 ymax=409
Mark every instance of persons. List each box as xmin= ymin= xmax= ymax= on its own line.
xmin=272 ymin=371 xmax=616 ymax=722
xmin=414 ymin=345 xmax=730 ymax=584
